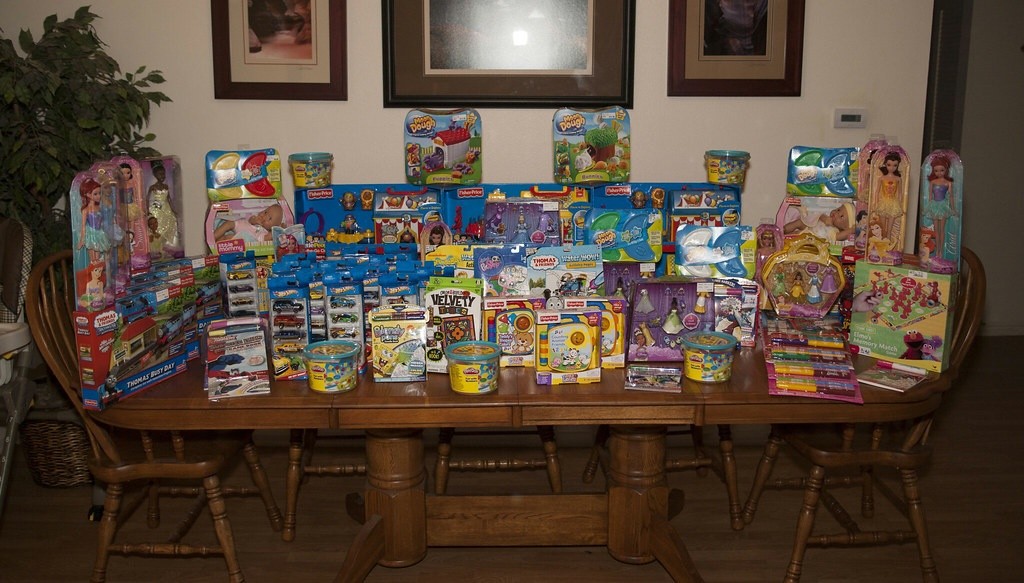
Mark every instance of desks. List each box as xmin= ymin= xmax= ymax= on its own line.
xmin=87 ymin=336 xmax=949 ymax=583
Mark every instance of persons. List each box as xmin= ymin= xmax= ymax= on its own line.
xmin=75 ymin=160 xmax=287 ymax=308
xmin=762 ymin=147 xmax=958 ymax=318
xmin=428 ymin=205 xmax=563 ymax=245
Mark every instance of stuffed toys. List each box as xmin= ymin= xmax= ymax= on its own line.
xmin=896 ymin=330 xmax=943 ymax=364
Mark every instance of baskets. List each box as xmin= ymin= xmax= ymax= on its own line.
xmin=21 ymin=406 xmax=90 ymax=487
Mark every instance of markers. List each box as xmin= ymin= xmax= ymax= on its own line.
xmin=770 ymin=330 xmax=857 ymax=395
xmin=208 ymin=324 xmax=262 ymax=336
xmin=876 ymin=358 xmax=930 ymax=376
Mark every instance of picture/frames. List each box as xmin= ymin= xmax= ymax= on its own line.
xmin=377 ymin=0 xmax=636 ymax=116
xmin=664 ymin=0 xmax=808 ymax=100
xmin=208 ymin=0 xmax=349 ymax=103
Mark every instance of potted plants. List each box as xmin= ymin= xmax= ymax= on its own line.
xmin=1 ymin=4 xmax=175 ymax=492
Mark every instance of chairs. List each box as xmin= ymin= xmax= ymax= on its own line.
xmin=24 ymin=247 xmax=284 ymax=583
xmin=740 ymin=247 xmax=986 ymax=583
xmin=279 ymin=426 xmax=367 ymax=544
xmin=433 ymin=427 xmax=565 ymax=497
xmin=582 ymin=421 xmax=745 ymax=533
xmin=0 ymin=216 xmax=35 ymax=515
